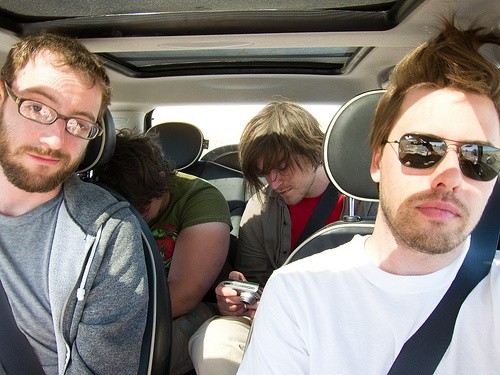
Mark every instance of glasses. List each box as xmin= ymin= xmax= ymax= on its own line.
xmin=386 ymin=133 xmax=500 ymax=182
xmin=4 ymin=81 xmax=104 ymax=139
xmin=138 ymin=206 xmax=151 ymax=217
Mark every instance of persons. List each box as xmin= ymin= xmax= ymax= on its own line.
xmin=237 ymin=18 xmax=500 ymax=374
xmin=186 ymin=100 xmax=380 ymax=374
xmin=88 ymin=130 xmax=233 ymax=375
xmin=400 ymin=135 xmax=483 ymax=181
xmin=1 ymin=33 xmax=151 ymax=374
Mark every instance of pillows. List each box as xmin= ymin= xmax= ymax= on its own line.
xmin=190 ymin=161 xmax=245 ymax=304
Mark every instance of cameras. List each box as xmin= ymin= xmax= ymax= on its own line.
xmin=223 ymin=280 xmax=263 ymax=306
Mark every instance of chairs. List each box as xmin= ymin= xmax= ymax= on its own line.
xmin=283 ymin=89 xmax=388 ymax=267
xmin=76 ymin=107 xmax=173 ymax=375
xmin=145 ymin=121 xmax=209 ymax=171
